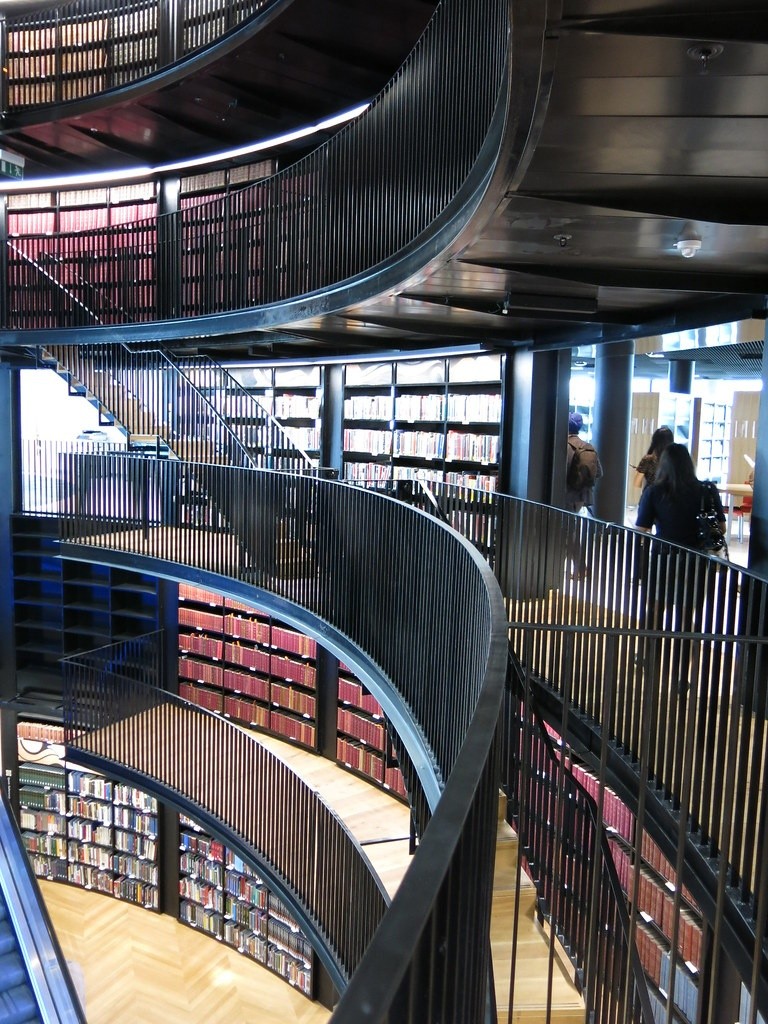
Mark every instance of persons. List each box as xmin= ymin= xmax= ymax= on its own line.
xmin=634 ymin=429 xmax=726 ymax=692
xmin=566 ymin=412 xmax=603 ymax=580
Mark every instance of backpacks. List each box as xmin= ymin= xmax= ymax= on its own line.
xmin=569 ymin=441 xmax=598 ymax=489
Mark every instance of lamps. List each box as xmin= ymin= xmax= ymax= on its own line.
xmin=675 ymin=240 xmax=704 ymax=259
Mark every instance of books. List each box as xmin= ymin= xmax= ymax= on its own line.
xmin=6 ymin=160 xmax=320 ymax=329
xmin=176 ymin=581 xmax=408 ymax=800
xmin=661 ymin=398 xmax=732 ymax=506
xmin=512 ymin=700 xmax=768 ymax=1024
xmin=15 ymin=719 xmax=311 ymax=996
xmin=0 ymin=0 xmax=268 ymax=106
xmin=129 ymin=393 xmax=500 ymax=567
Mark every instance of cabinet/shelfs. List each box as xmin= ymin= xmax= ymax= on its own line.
xmin=0 ymin=0 xmax=768 ymax=1024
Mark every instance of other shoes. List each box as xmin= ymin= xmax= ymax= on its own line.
xmin=633 ymin=652 xmax=648 ymax=666
xmin=570 ymin=567 xmax=588 ymax=579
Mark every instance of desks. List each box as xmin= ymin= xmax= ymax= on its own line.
xmin=714 ymin=484 xmax=753 ymax=544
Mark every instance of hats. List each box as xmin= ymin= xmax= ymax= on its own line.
xmin=569 ymin=413 xmax=583 ymax=431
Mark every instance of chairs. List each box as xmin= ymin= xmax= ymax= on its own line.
xmin=738 ymin=481 xmax=752 ymax=540
xmin=712 ymin=481 xmax=743 ymax=543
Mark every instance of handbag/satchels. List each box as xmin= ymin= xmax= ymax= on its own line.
xmin=695 ymin=481 xmax=725 ymax=551
